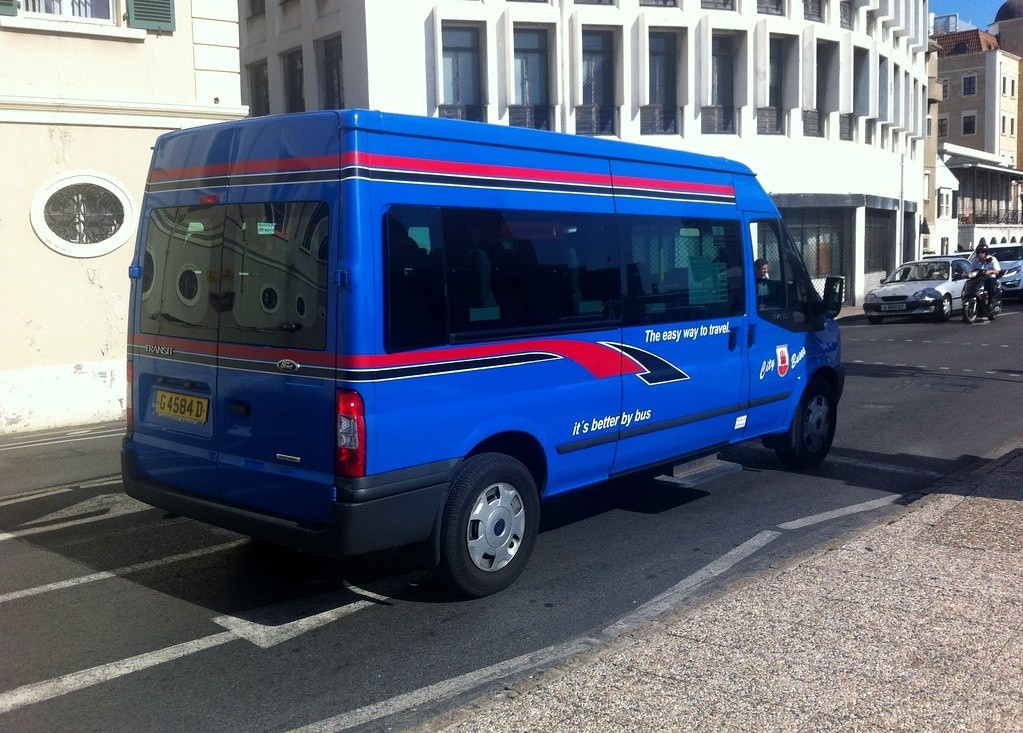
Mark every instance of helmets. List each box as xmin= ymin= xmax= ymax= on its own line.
xmin=976 ymin=244 xmax=988 ymax=255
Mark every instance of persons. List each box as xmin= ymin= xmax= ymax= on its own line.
xmin=755 ymin=259 xmax=769 ymax=279
xmin=937 ymin=265 xmax=948 ymax=278
xmin=965 ymin=244 xmax=1000 ymax=306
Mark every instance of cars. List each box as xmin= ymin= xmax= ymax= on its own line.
xmin=863 ymin=257 xmax=970 ymax=323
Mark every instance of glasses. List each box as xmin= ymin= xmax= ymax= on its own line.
xmin=978 ymin=252 xmax=985 ymax=254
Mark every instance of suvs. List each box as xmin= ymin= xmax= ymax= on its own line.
xmin=965 ymin=243 xmax=1023 ymax=303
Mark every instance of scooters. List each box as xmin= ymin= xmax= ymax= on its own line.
xmin=962 ymin=258 xmax=1002 ymax=324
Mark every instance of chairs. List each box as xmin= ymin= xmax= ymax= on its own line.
xmin=386 ymin=233 xmax=729 ymax=351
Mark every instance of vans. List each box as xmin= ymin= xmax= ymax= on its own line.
xmin=120 ymin=108 xmax=845 ymax=603
